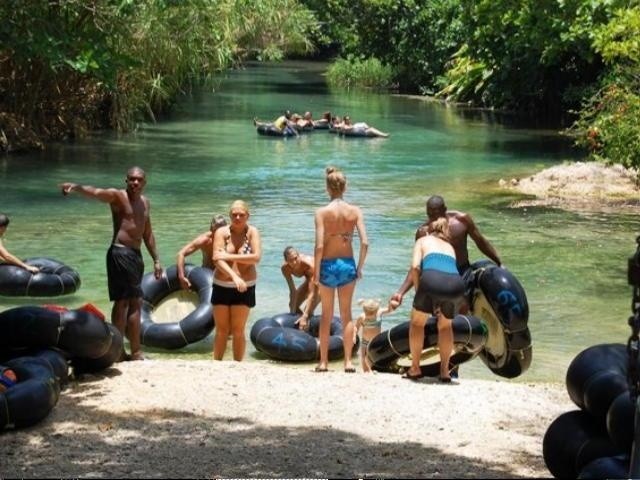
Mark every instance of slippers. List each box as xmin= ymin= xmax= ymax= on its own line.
xmin=438 ymin=375 xmax=451 ymax=382
xmin=402 ymin=371 xmax=425 ymax=378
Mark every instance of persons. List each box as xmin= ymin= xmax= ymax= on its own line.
xmin=281 ymin=246 xmax=320 ymax=332
xmin=401 ymin=217 xmax=464 ymax=382
xmin=352 ymin=297 xmax=399 ymax=372
xmin=312 ymin=166 xmax=368 ymax=373
xmin=176 ymin=214 xmax=227 ymax=291
xmin=57 ymin=165 xmax=162 ymax=362
xmin=210 ymin=199 xmax=262 ymax=360
xmin=388 ymin=194 xmax=505 ymax=376
xmin=253 ymin=109 xmax=390 ymax=139
xmin=0 ymin=213 xmax=40 ymax=274
